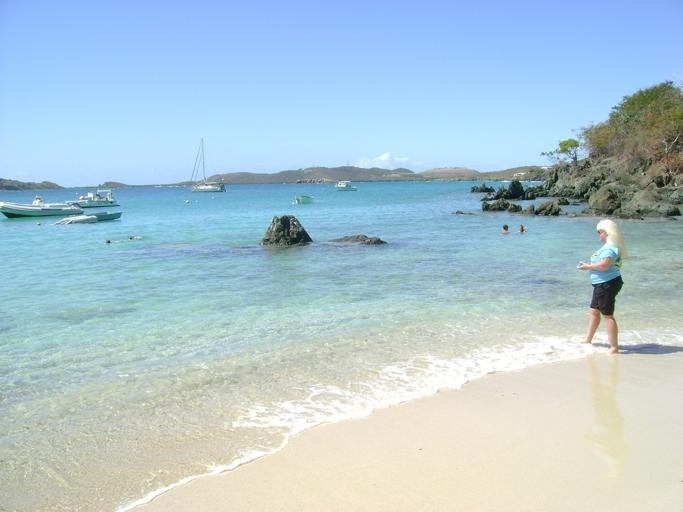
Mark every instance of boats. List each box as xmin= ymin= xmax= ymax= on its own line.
xmin=296 ymin=194 xmax=314 ymax=204
xmin=334 ymin=180 xmax=357 ymax=191
xmin=0 ymin=189 xmax=124 ymax=226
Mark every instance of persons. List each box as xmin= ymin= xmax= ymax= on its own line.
xmin=571 ymin=218 xmax=629 ymax=355
xmin=517 ymin=224 xmax=526 ymax=233
xmin=501 ymin=225 xmax=510 ymax=236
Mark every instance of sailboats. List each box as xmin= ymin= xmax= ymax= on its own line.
xmin=188 ymin=137 xmax=227 ymax=192
